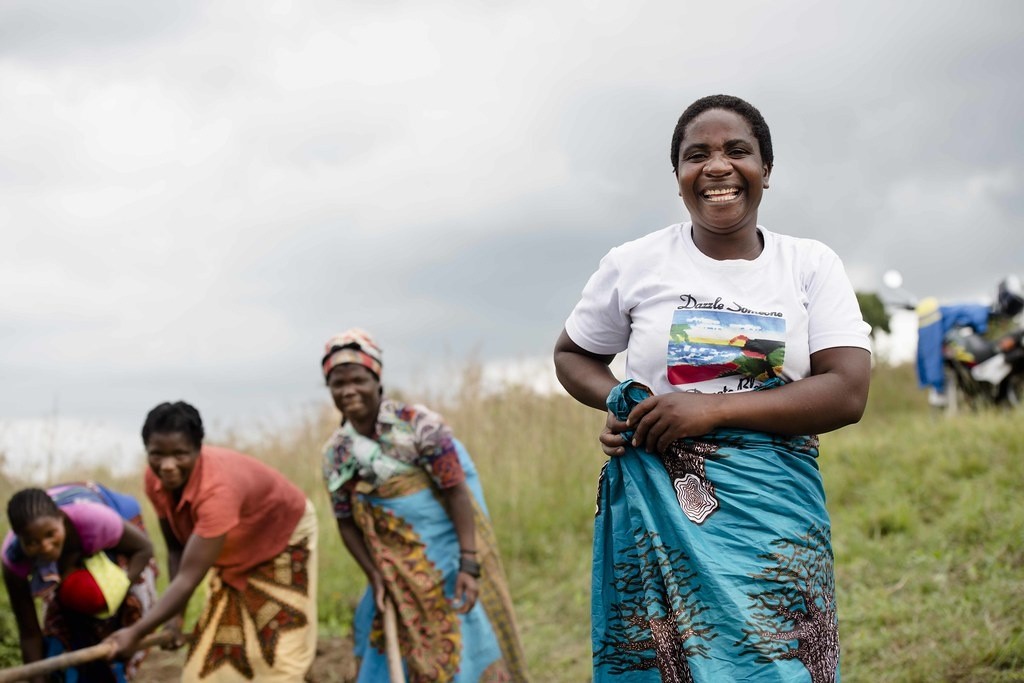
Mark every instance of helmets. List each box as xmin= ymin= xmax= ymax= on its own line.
xmin=999 ymin=274 xmax=1024 ymax=314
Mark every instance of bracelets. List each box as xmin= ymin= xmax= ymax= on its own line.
xmin=457 ymin=549 xmax=482 ymax=579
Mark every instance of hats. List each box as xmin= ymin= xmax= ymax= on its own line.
xmin=322 ymin=328 xmax=383 ymax=378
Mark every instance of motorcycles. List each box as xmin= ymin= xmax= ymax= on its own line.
xmin=880 ymin=271 xmax=1024 ymax=409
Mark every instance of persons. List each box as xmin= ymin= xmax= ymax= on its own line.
xmin=554 ymin=94 xmax=871 ymax=683
xmin=0 ymin=479 xmax=160 ymax=683
xmin=319 ymin=331 xmax=534 ymax=683
xmin=101 ymin=401 xmax=319 ymax=683
